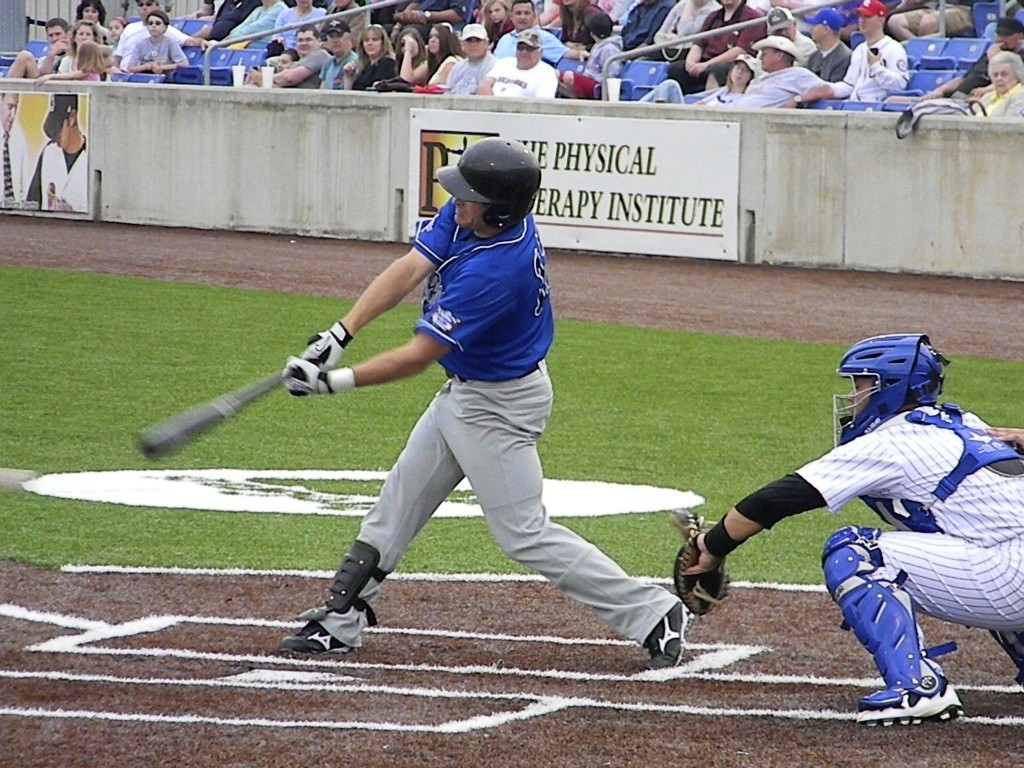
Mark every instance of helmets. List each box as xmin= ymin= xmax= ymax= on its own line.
xmin=830 ymin=335 xmax=942 ymax=448
xmin=436 ymin=136 xmax=542 ymax=226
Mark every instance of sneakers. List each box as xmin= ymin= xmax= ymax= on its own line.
xmin=281 ymin=621 xmax=354 ymax=654
xmin=857 ymin=662 xmax=965 ymax=727
xmin=642 ymin=601 xmax=689 ymax=672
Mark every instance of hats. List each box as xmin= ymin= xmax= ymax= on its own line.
xmin=733 ymin=53 xmax=758 ymax=77
xmin=803 ymin=6 xmax=842 ymax=29
xmin=751 ymin=34 xmax=804 ymax=63
xmin=856 ymin=0 xmax=887 ymax=17
xmin=766 ymin=6 xmax=794 ymax=36
xmin=460 ymin=23 xmax=489 ymax=42
xmin=325 ymin=19 xmax=350 ymax=37
xmin=515 ymin=29 xmax=540 ymax=48
xmin=583 ymin=11 xmax=613 ymax=39
xmin=995 ymin=17 xmax=1023 ymax=34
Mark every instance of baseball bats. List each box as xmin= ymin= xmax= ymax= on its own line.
xmin=133 ymin=367 xmax=296 ymax=462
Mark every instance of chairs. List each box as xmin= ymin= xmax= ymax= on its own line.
xmin=0 ymin=0 xmax=1024 ymax=112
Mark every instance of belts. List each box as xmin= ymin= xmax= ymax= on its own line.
xmin=443 ymin=367 xmax=542 ymax=386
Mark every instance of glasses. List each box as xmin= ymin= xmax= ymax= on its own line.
xmin=149 ymin=21 xmax=161 ymax=25
xmin=517 ymin=45 xmax=534 ymax=52
xmin=138 ymin=2 xmax=152 ymax=6
xmin=735 ymin=64 xmax=749 ymax=75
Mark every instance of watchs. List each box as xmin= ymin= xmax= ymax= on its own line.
xmin=794 ymin=95 xmax=804 ymax=108
xmin=425 ymin=11 xmax=431 ymax=21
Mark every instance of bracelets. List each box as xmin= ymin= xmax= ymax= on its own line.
xmin=327 ymin=368 xmax=356 ymax=392
xmin=704 ymin=514 xmax=749 ymax=558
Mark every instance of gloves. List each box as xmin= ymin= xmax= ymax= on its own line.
xmin=281 ymin=355 xmax=335 ymax=398
xmin=302 ymin=322 xmax=353 ymax=371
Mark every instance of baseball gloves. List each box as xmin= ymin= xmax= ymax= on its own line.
xmin=667 ymin=506 xmax=730 ymax=617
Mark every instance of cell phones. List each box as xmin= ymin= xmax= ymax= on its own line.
xmin=870 ymin=48 xmax=879 ymax=55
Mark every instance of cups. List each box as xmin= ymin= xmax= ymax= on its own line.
xmin=261 ymin=67 xmax=275 ymax=90
xmin=607 ymin=78 xmax=620 ymax=101
xmin=232 ymin=65 xmax=246 ymax=86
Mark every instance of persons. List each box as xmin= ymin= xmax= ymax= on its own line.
xmin=477 ymin=30 xmax=559 ymax=98
xmin=26 ymin=94 xmax=87 ymax=211
xmin=190 ymin=0 xmax=678 ymax=94
xmin=127 ymin=10 xmax=189 ymax=75
xmin=668 ymin=0 xmax=767 ymax=96
xmin=964 ymin=51 xmax=1024 ymax=117
xmin=737 ymin=36 xmax=834 ymax=108
xmin=803 ymin=8 xmax=852 ymax=83
xmin=756 ymin=6 xmax=818 ymax=75
xmin=277 ymin=138 xmax=693 ymax=670
xmin=832 ymin=0 xmax=909 ymax=104
xmin=113 ymin=0 xmax=210 ymax=68
xmin=918 ymin=18 xmax=1024 ymax=101
xmin=647 ymin=0 xmax=723 ymax=65
xmin=5 ymin=0 xmax=129 ymax=86
xmin=694 ymin=54 xmax=761 ymax=106
xmin=888 ymin=0 xmax=996 ymax=42
xmin=0 ymin=94 xmax=33 ymax=203
xmin=673 ymin=334 xmax=1024 ymax=728
